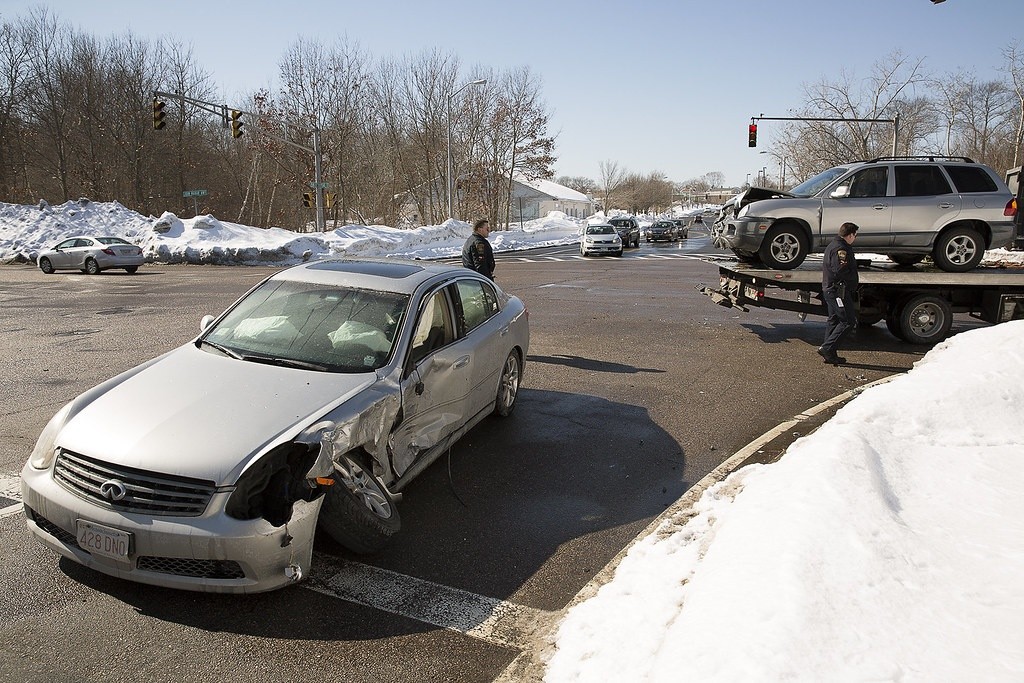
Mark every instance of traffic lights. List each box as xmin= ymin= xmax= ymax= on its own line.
xmin=327 ymin=193 xmax=338 ymax=207
xmin=232 ymin=110 xmax=244 ymax=138
xmin=749 ymin=125 xmax=758 ymax=147
xmin=153 ymin=100 xmax=167 ymax=130
xmin=303 ymin=193 xmax=313 ymax=208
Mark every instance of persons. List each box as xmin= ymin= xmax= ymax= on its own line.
xmin=463 ymin=219 xmax=495 ymax=283
xmin=817 ymin=223 xmax=860 ymax=365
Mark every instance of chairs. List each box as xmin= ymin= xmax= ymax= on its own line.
xmin=863 ymin=182 xmax=878 ymax=196
xmin=81 ymin=240 xmax=87 ymax=246
xmin=589 ymin=229 xmax=596 ymax=234
xmin=604 ymin=228 xmax=611 ymax=233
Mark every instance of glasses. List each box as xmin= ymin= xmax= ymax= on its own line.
xmin=851 ymin=234 xmax=857 ymax=238
xmin=477 ymin=227 xmax=489 ymax=231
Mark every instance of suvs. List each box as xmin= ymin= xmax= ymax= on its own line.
xmin=712 ymin=156 xmax=1018 ymax=274
xmin=607 ymin=215 xmax=640 ymax=248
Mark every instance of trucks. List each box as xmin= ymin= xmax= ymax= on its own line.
xmin=695 ymin=165 xmax=1024 ymax=347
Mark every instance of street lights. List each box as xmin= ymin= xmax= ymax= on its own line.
xmin=746 ymin=166 xmax=767 ymax=187
xmin=447 ymin=79 xmax=487 ymax=218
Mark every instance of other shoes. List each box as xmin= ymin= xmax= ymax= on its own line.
xmin=823 ymin=356 xmax=846 ymax=364
xmin=817 ymin=346 xmax=838 ymax=364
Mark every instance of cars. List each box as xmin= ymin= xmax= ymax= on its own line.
xmin=668 ymin=219 xmax=688 ymax=239
xmin=704 ymin=207 xmax=711 ymax=213
xmin=20 ymin=260 xmax=531 ymax=594
xmin=694 ymin=215 xmax=703 ymax=223
xmin=581 ymin=224 xmax=623 ymax=257
xmin=646 ymin=220 xmax=679 ymax=243
xmin=715 ymin=209 xmax=720 ymax=215
xmin=37 ymin=235 xmax=146 ymax=275
